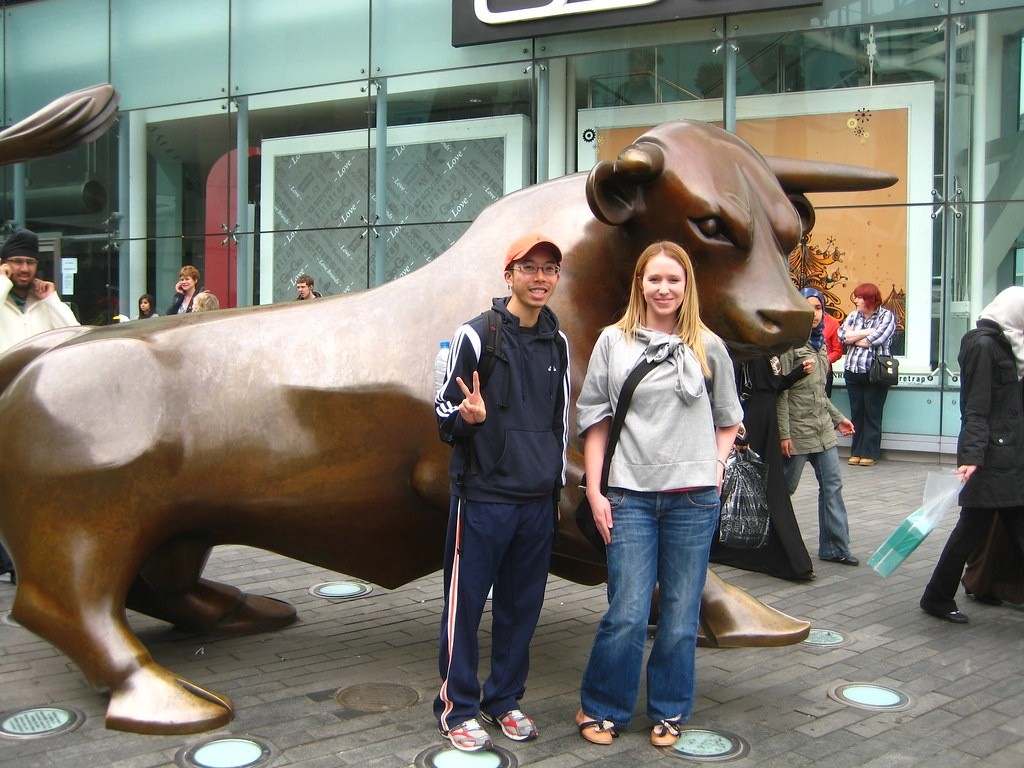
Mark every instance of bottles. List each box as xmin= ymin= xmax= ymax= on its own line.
xmin=434 ymin=342 xmax=450 ymax=396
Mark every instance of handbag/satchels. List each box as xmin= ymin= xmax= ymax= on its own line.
xmin=716 ymin=447 xmax=771 ymax=548
xmin=869 ymin=346 xmax=899 ymax=387
xmin=574 ymin=472 xmax=609 ymax=554
xmin=866 ymin=465 xmax=968 ymax=578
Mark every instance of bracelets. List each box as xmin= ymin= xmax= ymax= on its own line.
xmin=718 ymin=459 xmax=727 ymax=468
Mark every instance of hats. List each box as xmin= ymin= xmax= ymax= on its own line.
xmin=0 ymin=228 xmax=38 ymax=260
xmin=504 ymin=234 xmax=562 ymax=275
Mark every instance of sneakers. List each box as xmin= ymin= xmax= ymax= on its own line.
xmin=437 ymin=717 xmax=494 ymax=752
xmin=480 ymin=707 xmax=539 ymax=741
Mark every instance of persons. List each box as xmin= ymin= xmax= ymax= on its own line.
xmin=919 ymin=285 xmax=1024 ymax=625
xmin=138 ymin=295 xmax=158 ymax=318
xmin=709 ymin=282 xmax=899 ymax=583
xmin=0 ymin=230 xmax=82 ymax=583
xmin=434 ymin=234 xmax=572 ymax=752
xmin=295 ymin=275 xmax=321 ymax=300
xmin=166 ymin=265 xmax=220 ymax=316
xmin=574 ymin=241 xmax=744 ymax=748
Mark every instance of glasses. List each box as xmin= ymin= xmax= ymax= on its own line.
xmin=508 ymin=263 xmax=561 ymax=275
xmin=5 ymin=256 xmax=39 ymax=269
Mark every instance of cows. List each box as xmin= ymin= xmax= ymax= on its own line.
xmin=0 ymin=120 xmax=899 ymax=735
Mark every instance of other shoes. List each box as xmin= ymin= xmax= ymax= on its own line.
xmin=651 ymin=714 xmax=682 ymax=745
xmin=575 ymin=707 xmax=619 ymax=745
xmin=965 ymin=586 xmax=1003 ymax=606
xmin=0 ymin=565 xmax=16 ymax=583
xmin=859 ymin=458 xmax=877 ymax=465
xmin=1000 ymin=586 xmax=1024 ymax=609
xmin=819 ymin=549 xmax=861 ymax=566
xmin=942 ymin=605 xmax=968 ymax=623
xmin=848 ymin=456 xmax=860 ymax=465
